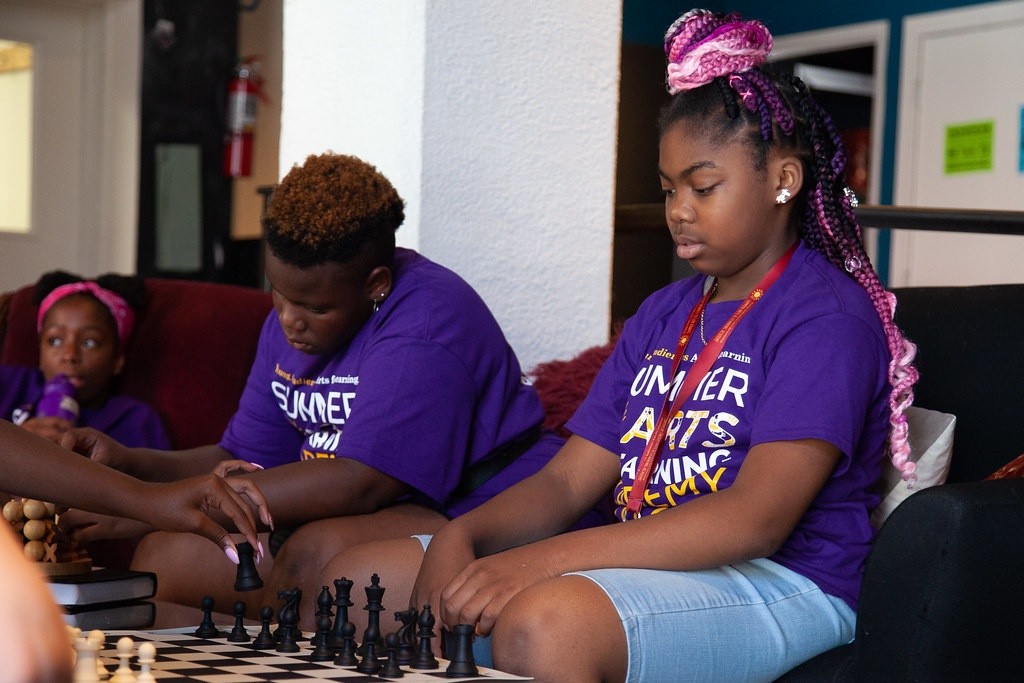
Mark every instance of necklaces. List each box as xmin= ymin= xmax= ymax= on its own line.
xmin=700 ymin=282 xmax=717 ymax=346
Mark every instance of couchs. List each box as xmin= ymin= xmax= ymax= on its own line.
xmin=1 ymin=281 xmax=1024 ymax=683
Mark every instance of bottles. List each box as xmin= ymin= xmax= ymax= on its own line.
xmin=37 ymin=374 xmax=80 ymax=422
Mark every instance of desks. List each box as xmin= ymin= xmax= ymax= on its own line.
xmin=46 ymin=591 xmax=542 ymax=683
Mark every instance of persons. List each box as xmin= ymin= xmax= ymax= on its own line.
xmin=319 ymin=4 xmax=919 ymax=683
xmin=0 ymin=513 xmax=74 ymax=683
xmin=0 ymin=419 xmax=275 ymax=566
xmin=50 ymin=152 xmax=567 ymax=643
xmin=0 ymin=270 xmax=171 ymax=448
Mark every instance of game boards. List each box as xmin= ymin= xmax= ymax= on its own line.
xmin=66 ymin=628 xmax=534 ymax=681
